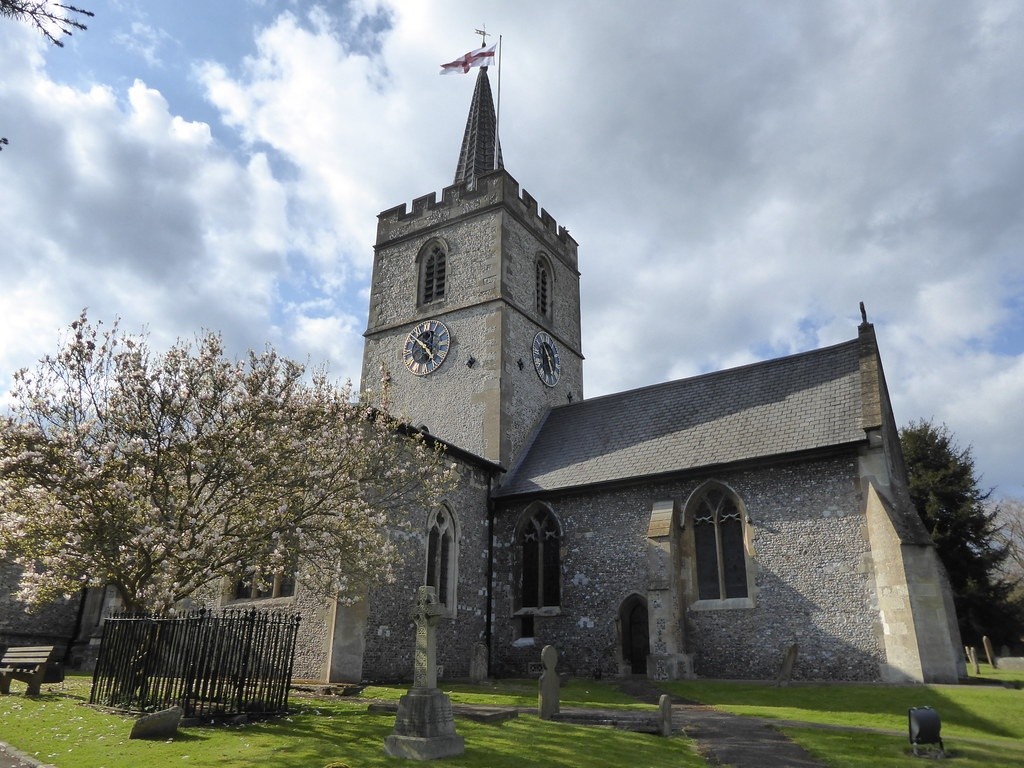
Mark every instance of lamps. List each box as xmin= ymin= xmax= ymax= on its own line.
xmin=908 ymin=706 xmax=945 ymax=759
xmin=42 ymin=662 xmax=65 ymax=691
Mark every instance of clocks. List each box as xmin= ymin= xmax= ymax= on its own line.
xmin=403 ymin=320 xmax=450 ymax=376
xmin=532 ymin=331 xmax=561 ymax=387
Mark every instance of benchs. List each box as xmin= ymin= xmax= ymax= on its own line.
xmin=0 ymin=646 xmax=55 ymax=696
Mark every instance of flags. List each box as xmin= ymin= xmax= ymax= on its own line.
xmin=438 ymin=40 xmax=498 ymax=76
